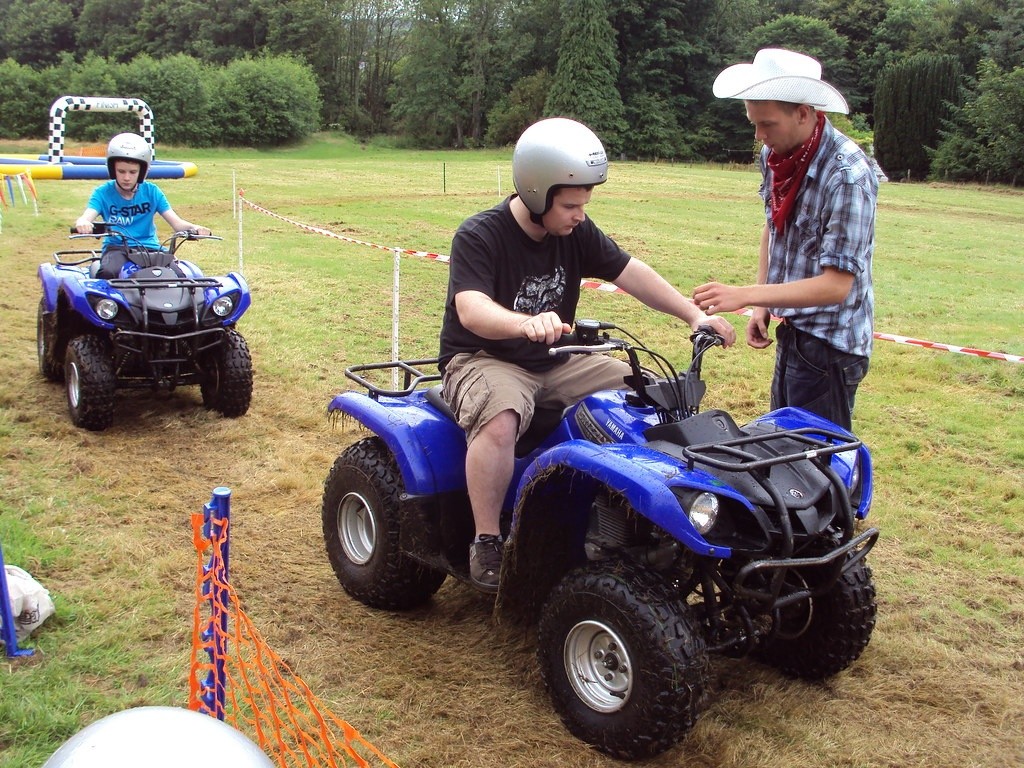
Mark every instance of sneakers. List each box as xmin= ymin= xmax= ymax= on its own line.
xmin=467 ymin=534 xmax=506 ymax=595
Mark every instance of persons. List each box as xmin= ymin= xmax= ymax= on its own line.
xmin=437 ymin=117 xmax=736 ymax=594
xmin=692 ymin=47 xmax=879 ymax=435
xmin=77 ymin=133 xmax=210 ymax=279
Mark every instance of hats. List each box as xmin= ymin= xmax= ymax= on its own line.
xmin=713 ymin=48 xmax=849 ymax=116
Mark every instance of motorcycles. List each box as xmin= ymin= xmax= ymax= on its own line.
xmin=35 ymin=221 xmax=255 ymax=434
xmin=320 ymin=319 xmax=880 ymax=762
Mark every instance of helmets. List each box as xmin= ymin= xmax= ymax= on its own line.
xmin=105 ymin=132 xmax=152 ymax=184
xmin=512 ymin=117 xmax=609 ymax=215
